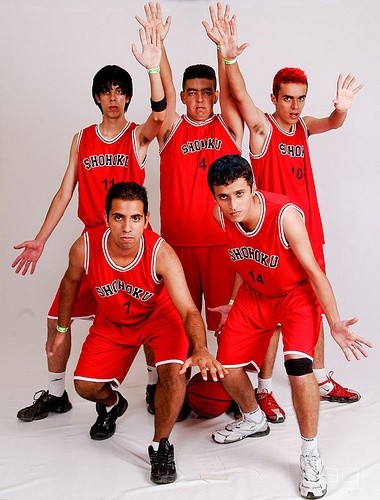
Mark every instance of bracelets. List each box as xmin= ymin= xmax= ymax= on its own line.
xmin=56 ymin=326 xmax=68 ymax=333
xmin=224 ymin=59 xmax=237 ymax=64
xmin=229 ymin=299 xmax=234 ymax=306
xmin=217 ymin=45 xmax=220 ymax=50
xmin=147 ymin=67 xmax=161 ymax=74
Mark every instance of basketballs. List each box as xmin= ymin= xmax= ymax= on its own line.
xmin=186 ymin=370 xmax=232 ymax=419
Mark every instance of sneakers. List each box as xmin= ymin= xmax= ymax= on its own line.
xmin=176 ymin=400 xmax=191 ymax=422
xmin=17 ymin=390 xmax=72 ymax=422
xmin=255 ymin=388 xmax=286 ymax=423
xmin=232 ymin=400 xmax=242 ymax=419
xmin=146 ymin=383 xmax=156 ymax=415
xmin=318 ymin=371 xmax=361 ymax=403
xmin=299 ymin=449 xmax=327 ymax=499
xmin=212 ymin=410 xmax=270 ymax=444
xmin=148 ymin=438 xmax=177 ymax=484
xmin=90 ymin=390 xmax=128 ymax=440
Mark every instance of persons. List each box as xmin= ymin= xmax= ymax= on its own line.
xmin=47 ymin=182 xmax=229 ymax=483
xmin=207 ymin=155 xmax=373 ymax=500
xmin=135 ymin=1 xmax=244 ymax=421
xmin=12 ymin=25 xmax=170 ymax=422
xmin=209 ymin=18 xmax=363 ymax=424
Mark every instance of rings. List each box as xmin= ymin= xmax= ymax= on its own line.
xmin=27 ymin=262 xmax=31 ymax=263
